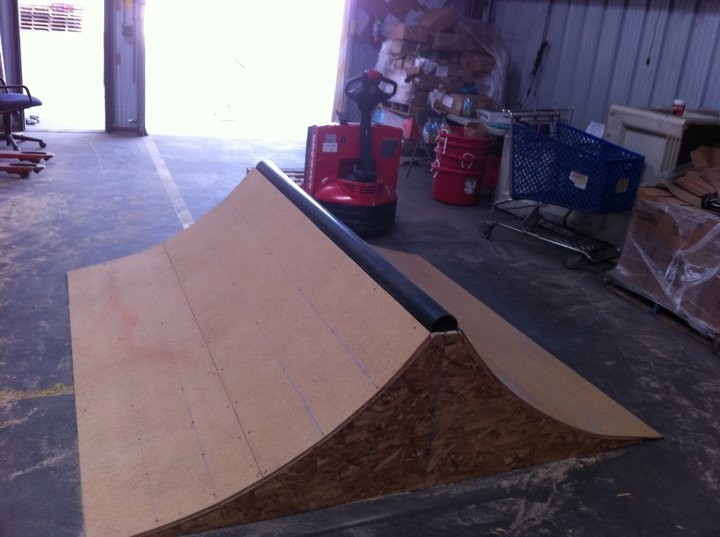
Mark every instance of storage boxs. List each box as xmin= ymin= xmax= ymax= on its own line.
xmin=608 ymin=142 xmax=719 ymax=341
xmin=372 ymin=8 xmax=530 ymax=204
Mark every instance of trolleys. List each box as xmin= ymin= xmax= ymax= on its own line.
xmin=479 ymin=108 xmax=645 ymax=269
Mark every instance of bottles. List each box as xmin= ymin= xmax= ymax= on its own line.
xmin=425 ymin=119 xmax=448 ymax=144
xmin=464 ymin=95 xmax=473 ymax=117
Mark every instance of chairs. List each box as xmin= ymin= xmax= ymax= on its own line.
xmin=0 ymin=78 xmax=46 ymax=152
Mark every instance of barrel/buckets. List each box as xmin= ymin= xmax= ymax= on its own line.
xmin=438 ymin=126 xmax=491 ymax=145
xmin=434 ymin=148 xmax=489 ymax=170
xmin=435 ymin=137 xmax=490 ymax=157
xmin=464 ymin=122 xmax=489 ymax=137
xmin=430 ymin=162 xmax=486 ymax=205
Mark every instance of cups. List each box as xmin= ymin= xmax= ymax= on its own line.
xmin=672 ymin=99 xmax=686 ymax=117
